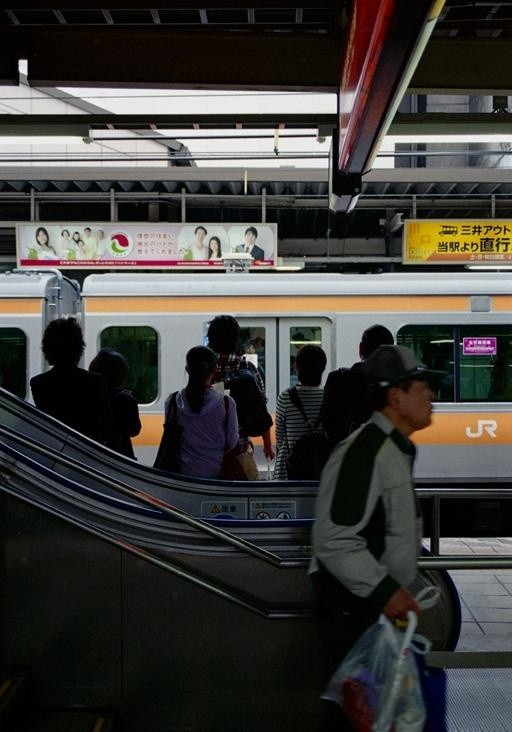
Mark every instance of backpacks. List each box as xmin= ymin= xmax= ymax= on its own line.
xmin=284 ymin=386 xmax=331 ymax=480
xmin=223 ymin=359 xmax=273 ymax=437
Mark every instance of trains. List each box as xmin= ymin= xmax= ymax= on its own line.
xmin=0 ymin=267 xmax=512 ymax=490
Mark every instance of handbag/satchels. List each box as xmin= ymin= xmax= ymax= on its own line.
xmin=154 ymin=391 xmax=184 ymax=471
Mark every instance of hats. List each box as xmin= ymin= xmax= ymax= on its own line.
xmin=361 ymin=344 xmax=448 ymax=391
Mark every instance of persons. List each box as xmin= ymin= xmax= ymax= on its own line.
xmin=303 ymin=339 xmax=453 ymax=731
xmin=30 ymin=317 xmax=141 ymax=462
xmin=165 ymin=315 xmax=276 ymax=481
xmin=271 ymin=325 xmax=394 ymax=481
xmin=20 ymin=223 xmax=275 ymax=263
